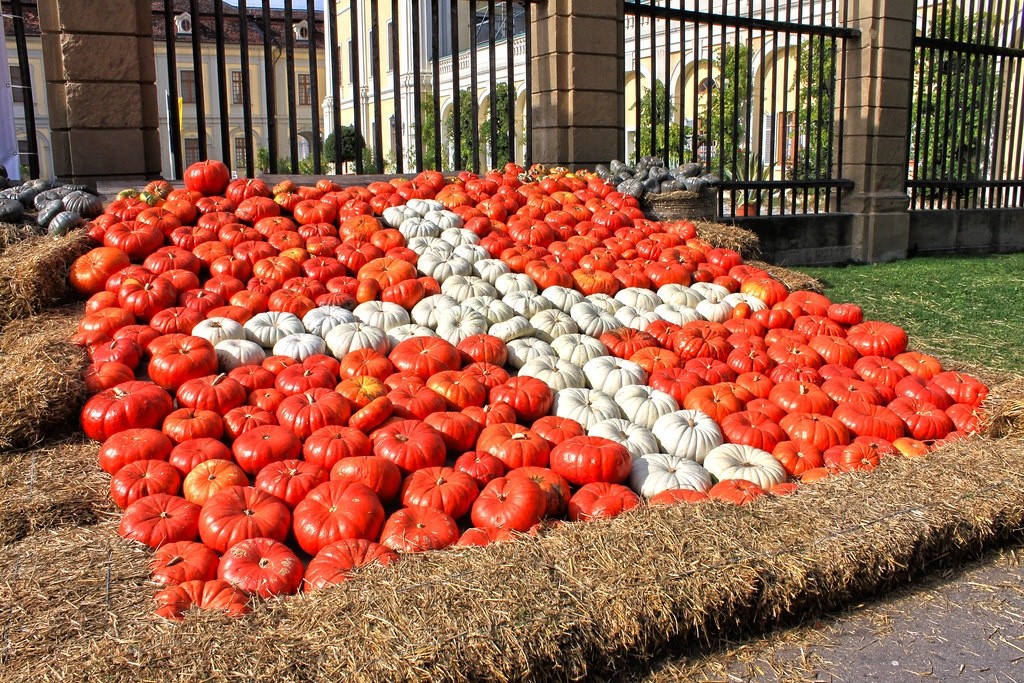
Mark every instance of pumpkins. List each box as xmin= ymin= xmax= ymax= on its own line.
xmin=67 ymin=156 xmax=987 ymax=619
xmin=0 ymin=164 xmax=102 ymax=236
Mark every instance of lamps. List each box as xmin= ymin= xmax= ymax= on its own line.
xmin=389 ymin=115 xmax=404 ymax=129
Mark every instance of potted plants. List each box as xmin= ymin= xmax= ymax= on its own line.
xmin=724 ymin=151 xmax=788 ymax=216
